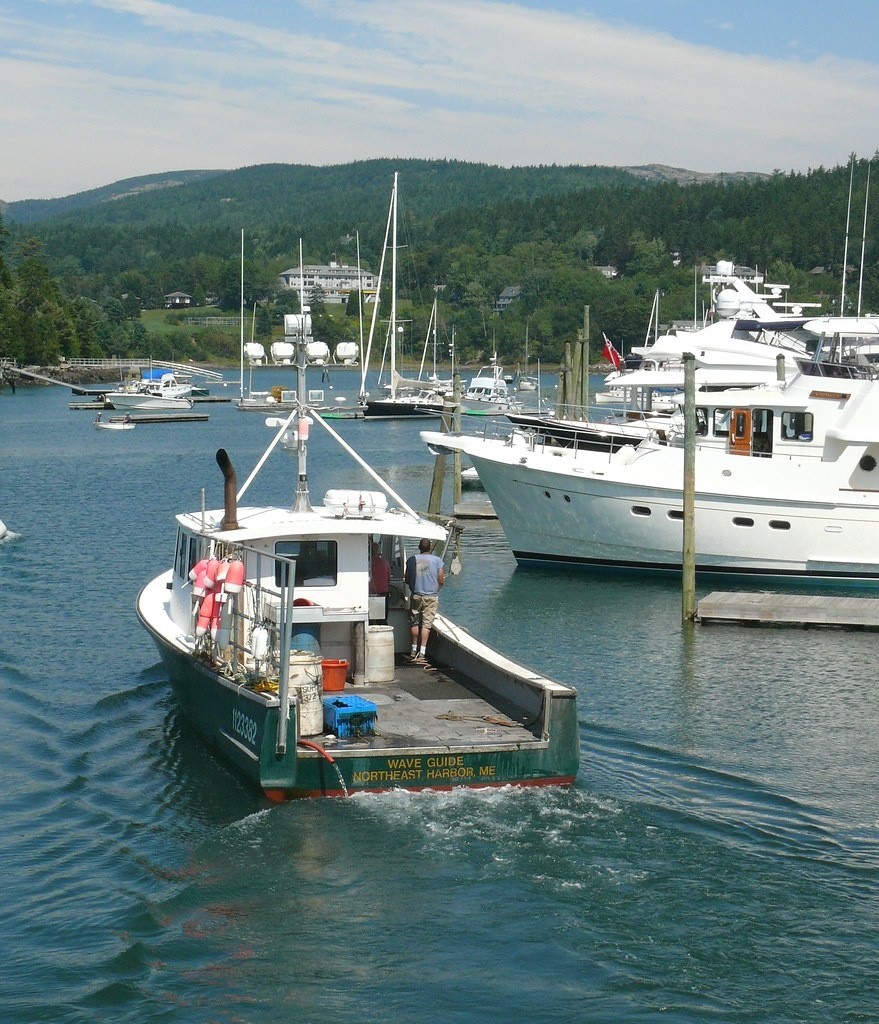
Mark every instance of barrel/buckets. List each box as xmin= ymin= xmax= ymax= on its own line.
xmin=290 ymin=657 xmax=323 ymax=736
xmin=367 ymin=625 xmax=394 ymax=682
xmin=321 ymin=659 xmax=348 ymax=690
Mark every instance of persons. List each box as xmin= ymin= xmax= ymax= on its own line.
xmin=95 ymin=412 xmax=101 ymax=422
xmin=369 ymin=543 xmax=391 ymax=625
xmin=357 ymin=395 xmax=363 ymax=406
xmin=123 ymin=412 xmax=131 ymax=423
xmin=404 ymin=538 xmax=445 ymax=659
xmin=99 ymin=395 xmax=104 ymax=401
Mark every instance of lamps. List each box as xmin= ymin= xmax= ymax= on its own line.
xmin=271 ymin=342 xmax=297 ymax=366
xmin=332 ymin=342 xmax=360 ymax=367
xmin=244 ymin=343 xmax=268 ymax=366
xmin=280 ymin=391 xmax=296 ymax=403
xmin=309 ymin=389 xmax=325 ymax=406
xmin=305 ymin=341 xmax=331 ymax=366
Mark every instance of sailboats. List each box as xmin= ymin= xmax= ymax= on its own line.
xmin=517 ymin=325 xmax=539 ymax=391
xmin=357 ymin=169 xmax=462 ymax=420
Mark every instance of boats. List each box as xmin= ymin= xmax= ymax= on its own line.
xmin=134 ymin=241 xmax=581 ymax=805
xmin=105 ymin=389 xmax=195 ymax=410
xmin=636 ymin=391 xmax=681 ymax=412
xmin=502 ymin=374 xmax=515 ymax=385
xmin=122 ymin=357 xmax=211 ymax=397
xmin=93 ymin=421 xmax=137 ymax=431
xmin=418 ymin=259 xmax=879 ymax=589
xmin=458 ymin=349 xmax=523 ymax=415
xmin=594 ymin=288 xmax=659 ymax=405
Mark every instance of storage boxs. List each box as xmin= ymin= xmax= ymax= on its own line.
xmin=322 ymin=694 xmax=378 ymax=739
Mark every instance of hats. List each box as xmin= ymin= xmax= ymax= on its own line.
xmin=368 ymin=542 xmax=382 ymax=556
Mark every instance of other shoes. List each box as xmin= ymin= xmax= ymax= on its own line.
xmin=409 ymin=650 xmax=417 ymax=658
xmin=418 ymin=653 xmax=425 ymax=659
